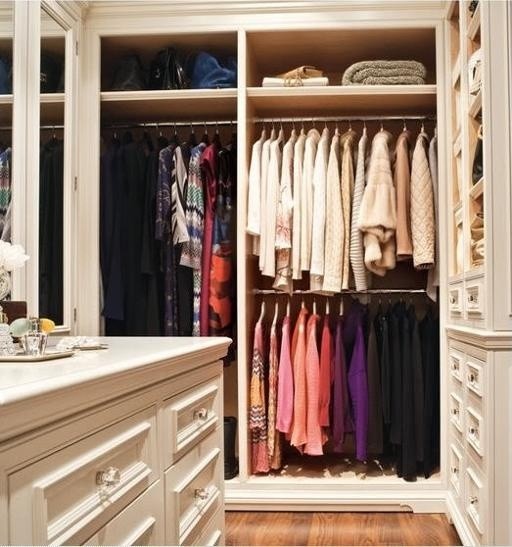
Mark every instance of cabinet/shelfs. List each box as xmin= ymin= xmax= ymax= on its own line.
xmin=445 ymin=3 xmax=512 ymax=547
xmin=0 ymin=335 xmax=231 ymax=546
xmin=0 ymin=1 xmax=447 ymax=515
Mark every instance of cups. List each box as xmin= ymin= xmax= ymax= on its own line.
xmin=21 ymin=318 xmax=46 ymax=354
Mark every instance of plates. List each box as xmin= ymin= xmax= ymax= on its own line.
xmin=0 ymin=352 xmax=75 ymax=362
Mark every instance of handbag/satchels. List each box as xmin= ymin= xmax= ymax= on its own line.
xmin=102 ymin=46 xmax=237 ymax=92
